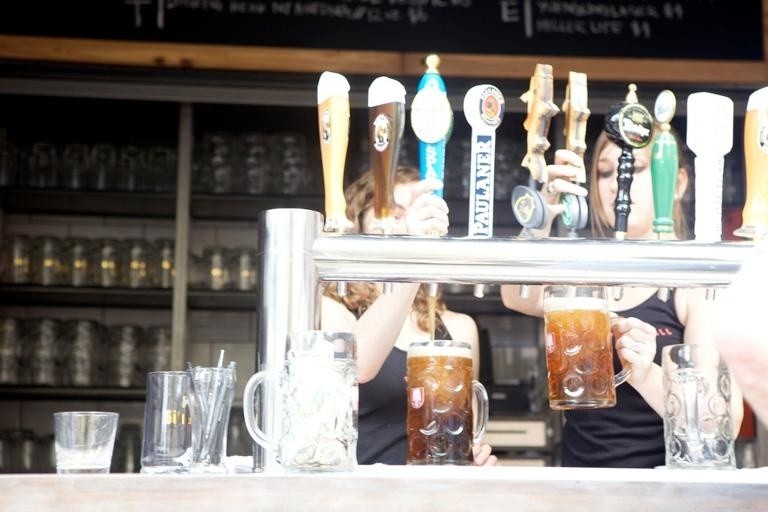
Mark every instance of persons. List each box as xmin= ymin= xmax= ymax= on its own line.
xmin=318 ymin=166 xmax=499 ymax=467
xmin=499 ymin=129 xmax=703 ymax=469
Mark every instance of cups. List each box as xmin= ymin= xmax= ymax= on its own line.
xmin=542 ymin=297 xmax=631 ymax=411
xmin=403 ymin=339 xmax=489 ymax=466
xmin=186 ymin=365 xmax=239 ymax=472
xmin=242 ymin=330 xmax=360 ymax=474
xmin=54 ymin=410 xmax=121 ymax=476
xmin=660 ymin=342 xmax=744 ymax=471
xmin=139 ymin=371 xmax=194 ymax=472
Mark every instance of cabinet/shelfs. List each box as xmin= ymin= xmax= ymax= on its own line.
xmin=0 ymin=80 xmax=767 ymax=474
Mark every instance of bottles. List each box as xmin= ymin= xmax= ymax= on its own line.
xmin=1 ymin=93 xmax=318 ymax=196
xmin=0 ymin=314 xmax=173 ymax=390
xmin=2 ymin=228 xmax=260 ymax=292
